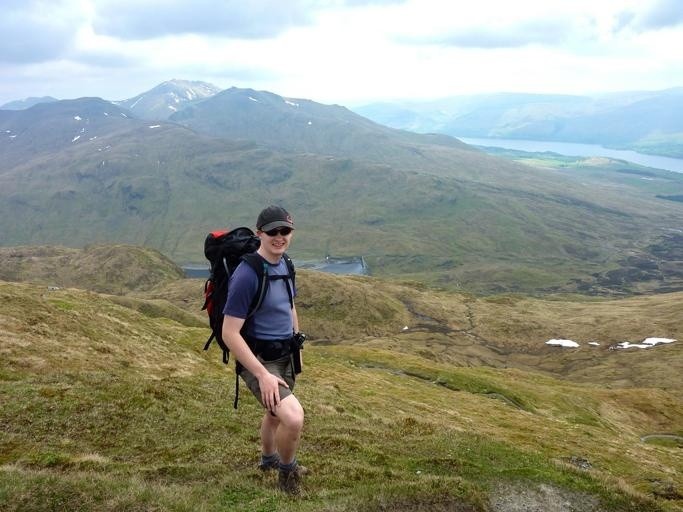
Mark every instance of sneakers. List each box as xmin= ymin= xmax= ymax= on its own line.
xmin=260 ymin=456 xmax=310 ymax=476
xmin=279 ymin=473 xmax=301 ymax=496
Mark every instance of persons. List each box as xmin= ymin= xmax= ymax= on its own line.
xmin=221 ymin=206 xmax=304 ymax=502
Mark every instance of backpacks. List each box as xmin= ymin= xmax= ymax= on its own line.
xmin=205 ymin=227 xmax=269 ymax=352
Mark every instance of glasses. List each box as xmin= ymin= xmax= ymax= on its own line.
xmin=265 ymin=228 xmax=291 ymax=236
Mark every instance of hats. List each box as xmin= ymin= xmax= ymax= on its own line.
xmin=257 ymin=207 xmax=295 ymax=232
